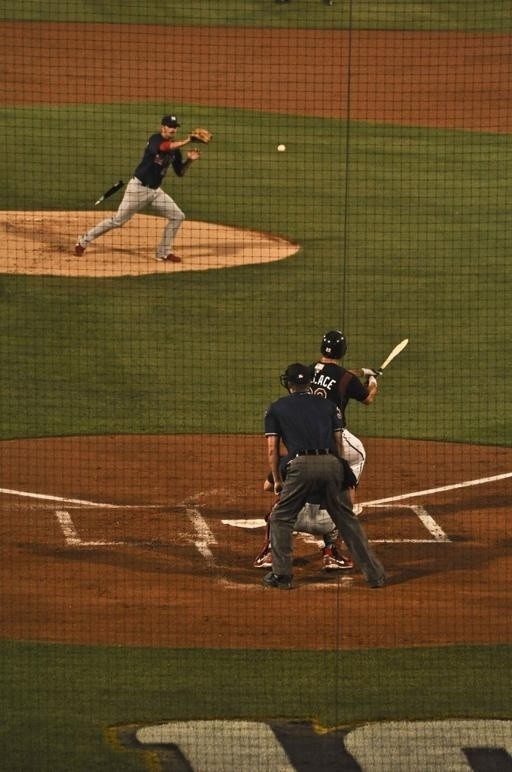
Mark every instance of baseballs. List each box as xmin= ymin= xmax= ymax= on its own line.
xmin=277 ymin=143 xmax=286 ymax=152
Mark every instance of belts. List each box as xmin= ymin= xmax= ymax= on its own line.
xmin=139 ymin=181 xmax=160 ymax=189
xmin=295 ymin=449 xmax=334 ymax=456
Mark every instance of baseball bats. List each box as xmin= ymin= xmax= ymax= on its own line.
xmin=375 ymin=339 xmax=409 ymax=381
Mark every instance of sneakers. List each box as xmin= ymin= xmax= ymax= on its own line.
xmin=263 ymin=571 xmax=292 ymax=589
xmin=75 ymin=234 xmax=86 ymax=256
xmin=322 ymin=547 xmax=354 ymax=571
xmin=162 ymin=253 xmax=181 ymax=263
xmin=253 ymin=548 xmax=275 ymax=568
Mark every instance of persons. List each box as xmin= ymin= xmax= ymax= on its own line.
xmin=251 ymin=448 xmax=355 ymax=571
xmin=305 ymin=330 xmax=378 ymax=516
xmin=260 ymin=360 xmax=389 ymax=588
xmin=74 ymin=112 xmax=201 ymax=264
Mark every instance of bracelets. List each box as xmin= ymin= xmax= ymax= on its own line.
xmin=362 ymin=367 xmax=377 ymax=378
xmin=368 ymin=375 xmax=377 ymax=388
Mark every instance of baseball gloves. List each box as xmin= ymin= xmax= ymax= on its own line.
xmin=188 ymin=125 xmax=212 ymax=147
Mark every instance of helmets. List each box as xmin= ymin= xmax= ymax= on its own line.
xmin=320 ymin=329 xmax=348 ymax=358
xmin=284 ymin=363 xmax=310 ymax=385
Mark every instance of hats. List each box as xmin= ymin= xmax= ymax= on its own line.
xmin=161 ymin=113 xmax=181 ymax=128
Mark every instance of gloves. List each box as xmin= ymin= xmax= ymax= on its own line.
xmin=361 ymin=367 xmax=383 ymax=387
